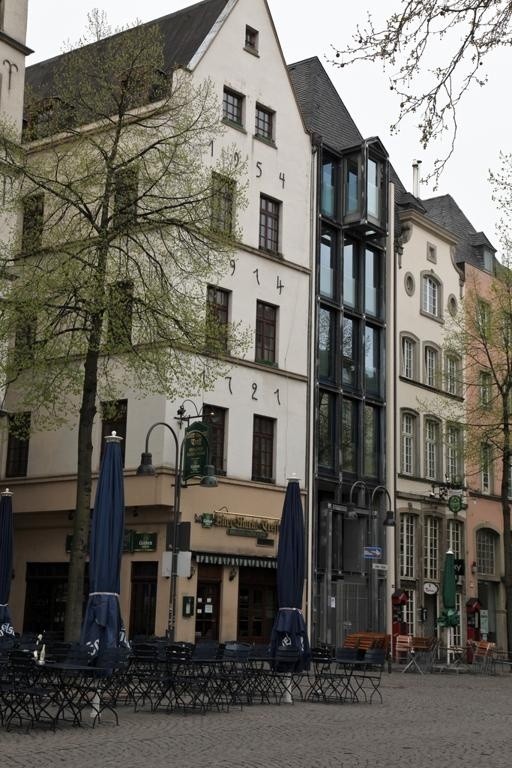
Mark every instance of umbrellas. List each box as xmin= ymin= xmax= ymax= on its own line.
xmin=436 ymin=546 xmax=460 ymax=665
xmin=1 ymin=487 xmax=18 ymax=637
xmin=78 ymin=429 xmax=132 ymax=716
xmin=267 ymin=472 xmax=313 ymax=703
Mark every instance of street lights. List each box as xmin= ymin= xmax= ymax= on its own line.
xmin=344 ymin=479 xmax=398 ymax=632
xmin=134 ymin=417 xmax=219 ymax=692
